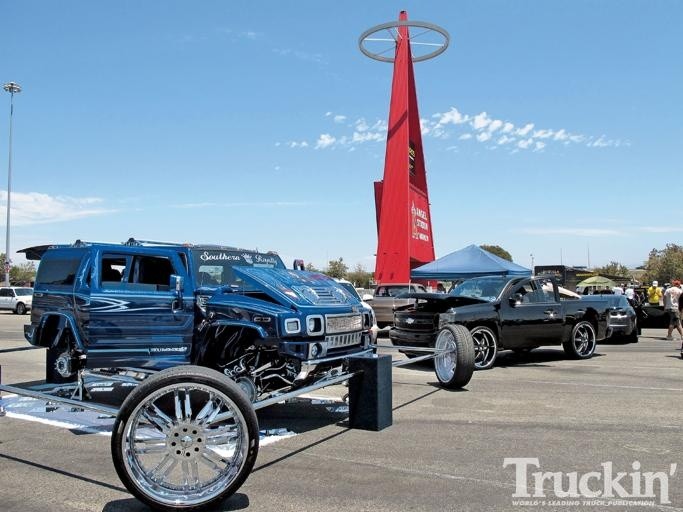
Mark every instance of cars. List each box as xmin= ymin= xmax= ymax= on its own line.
xmin=0 ymin=243 xmax=429 ymax=403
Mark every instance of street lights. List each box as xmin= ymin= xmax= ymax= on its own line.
xmin=3 ymin=83 xmax=22 ymax=286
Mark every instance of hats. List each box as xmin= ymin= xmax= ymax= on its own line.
xmin=652 ymin=281 xmax=658 ymax=287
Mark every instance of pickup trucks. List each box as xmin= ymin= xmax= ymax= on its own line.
xmin=389 ymin=274 xmax=638 ymax=370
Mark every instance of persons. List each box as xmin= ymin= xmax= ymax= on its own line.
xmin=583 ymin=279 xmax=683 ymax=359
